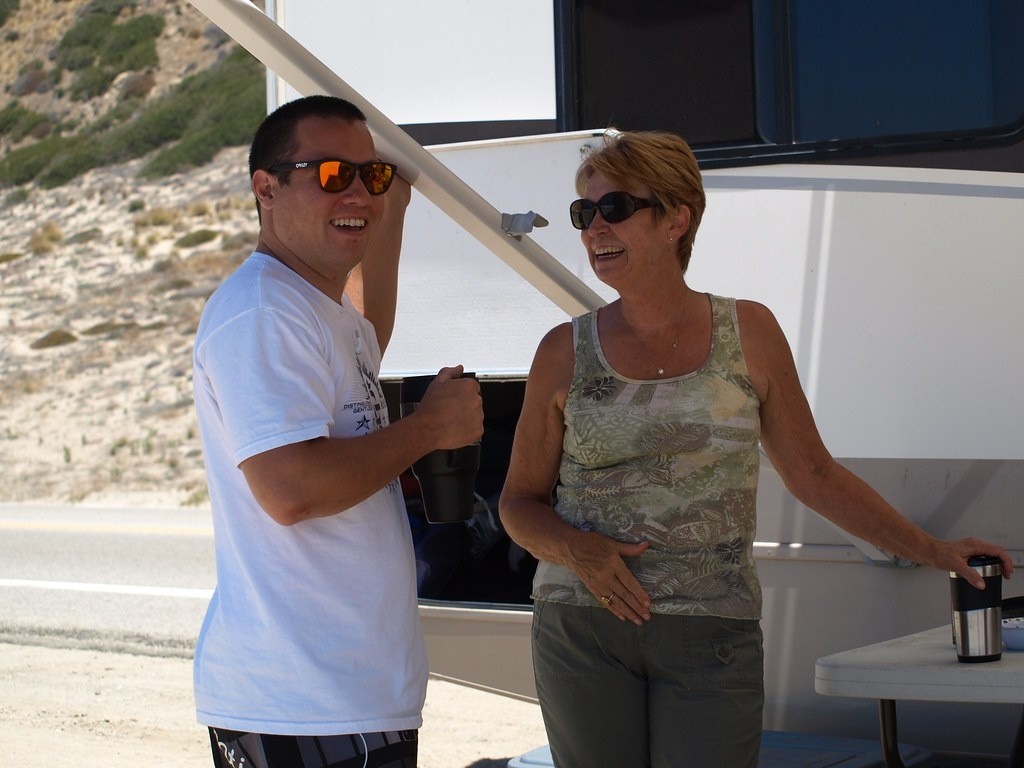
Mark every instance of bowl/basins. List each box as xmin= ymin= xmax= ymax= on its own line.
xmin=1001 ymin=617 xmax=1024 ymax=651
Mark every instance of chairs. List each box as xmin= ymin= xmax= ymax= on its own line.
xmin=572 ymin=0 xmax=1024 ymax=169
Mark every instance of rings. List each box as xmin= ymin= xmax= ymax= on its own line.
xmin=601 ymin=594 xmax=614 ymax=605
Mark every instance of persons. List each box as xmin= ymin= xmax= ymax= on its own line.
xmin=195 ymin=96 xmax=484 ymax=768
xmin=499 ymin=132 xmax=1014 ymax=768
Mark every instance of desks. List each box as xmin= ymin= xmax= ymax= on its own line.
xmin=815 ymin=623 xmax=1024 ymax=767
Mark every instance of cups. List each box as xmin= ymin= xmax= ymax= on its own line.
xmin=400 ymin=372 xmax=485 ymax=524
xmin=949 ymin=554 xmax=1002 ymax=663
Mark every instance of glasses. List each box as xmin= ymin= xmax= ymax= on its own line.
xmin=570 ymin=191 xmax=663 ymax=230
xmin=267 ymin=158 xmax=398 ymax=196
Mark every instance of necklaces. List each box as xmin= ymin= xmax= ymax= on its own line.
xmin=655 ymin=289 xmax=687 ymax=375
